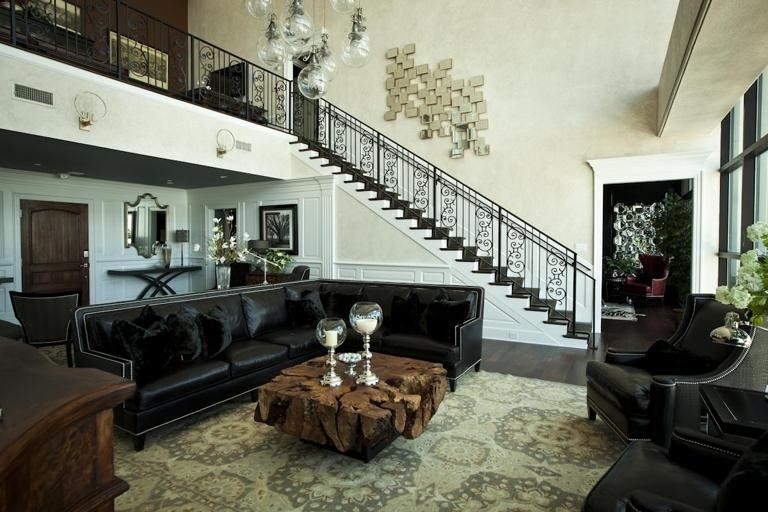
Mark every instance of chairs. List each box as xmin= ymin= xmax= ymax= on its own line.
xmin=579 ymin=426 xmax=768 ymax=512
xmin=10 ymin=289 xmax=82 ymax=368
xmin=585 ymin=293 xmax=768 ymax=446
xmin=625 ymin=254 xmax=674 ymax=299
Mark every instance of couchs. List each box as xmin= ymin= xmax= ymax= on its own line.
xmin=71 ymin=277 xmax=485 ymax=452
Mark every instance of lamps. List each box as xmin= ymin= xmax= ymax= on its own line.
xmin=245 ymin=0 xmax=370 ymax=101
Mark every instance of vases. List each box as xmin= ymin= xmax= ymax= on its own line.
xmin=215 ymin=265 xmax=232 ymax=290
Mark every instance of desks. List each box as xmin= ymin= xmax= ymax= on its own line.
xmin=108 ymin=265 xmax=202 ymax=301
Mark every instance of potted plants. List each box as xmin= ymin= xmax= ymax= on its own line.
xmin=603 ymin=254 xmax=639 ymax=302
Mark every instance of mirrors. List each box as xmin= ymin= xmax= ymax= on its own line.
xmin=124 ymin=192 xmax=170 ymax=259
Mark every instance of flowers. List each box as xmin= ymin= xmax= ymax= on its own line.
xmin=191 ymin=208 xmax=253 ymax=267
xmin=710 ymin=220 xmax=768 ymax=328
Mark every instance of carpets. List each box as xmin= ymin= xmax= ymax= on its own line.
xmin=37 ymin=343 xmax=626 ymax=512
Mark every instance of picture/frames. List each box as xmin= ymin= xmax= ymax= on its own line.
xmin=106 ymin=27 xmax=171 ymax=93
xmin=1 ymin=1 xmax=85 ymax=37
xmin=259 ymin=204 xmax=298 ymax=255
